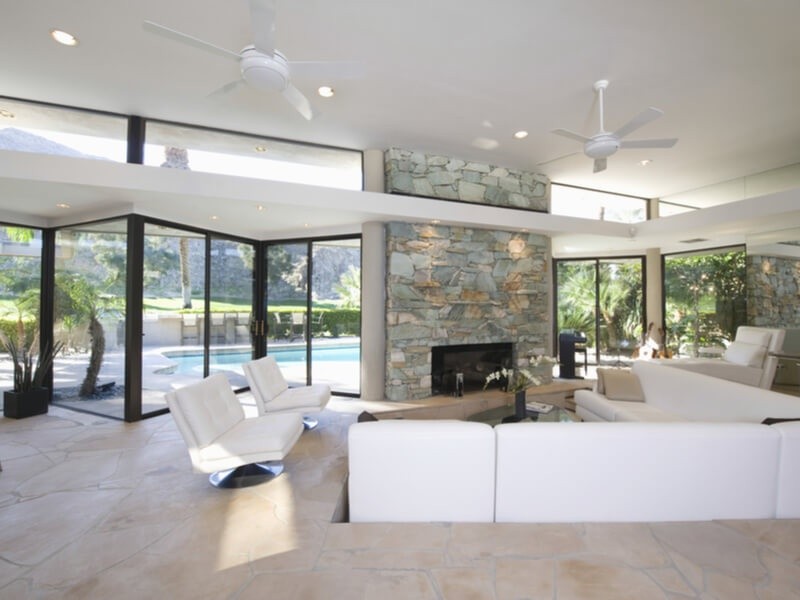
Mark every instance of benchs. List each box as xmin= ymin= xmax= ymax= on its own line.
xmin=157 ymin=366 xmax=308 ymax=488
xmin=242 ymin=354 xmax=332 ymax=432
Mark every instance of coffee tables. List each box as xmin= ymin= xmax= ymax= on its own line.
xmin=466 ymin=400 xmax=572 ymax=427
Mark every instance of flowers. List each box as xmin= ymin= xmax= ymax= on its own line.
xmin=483 ymin=366 xmax=542 ymax=398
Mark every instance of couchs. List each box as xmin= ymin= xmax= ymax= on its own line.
xmin=338 ymin=359 xmax=800 ymax=523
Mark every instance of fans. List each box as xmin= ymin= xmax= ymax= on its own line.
xmin=530 ymin=77 xmax=680 ymax=174
xmin=141 ymin=0 xmax=366 ymax=121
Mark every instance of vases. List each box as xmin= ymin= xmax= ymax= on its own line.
xmin=516 ymin=386 xmax=526 ymax=418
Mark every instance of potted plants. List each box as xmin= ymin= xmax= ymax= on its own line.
xmin=2 ymin=333 xmax=70 ymax=421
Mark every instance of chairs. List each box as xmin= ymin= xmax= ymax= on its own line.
xmin=662 ymin=321 xmax=788 ymax=389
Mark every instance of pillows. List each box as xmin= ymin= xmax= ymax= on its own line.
xmin=722 ymin=341 xmax=764 ymax=367
xmin=595 ymin=367 xmax=630 ymax=393
xmin=601 ymin=368 xmax=638 ymax=402
